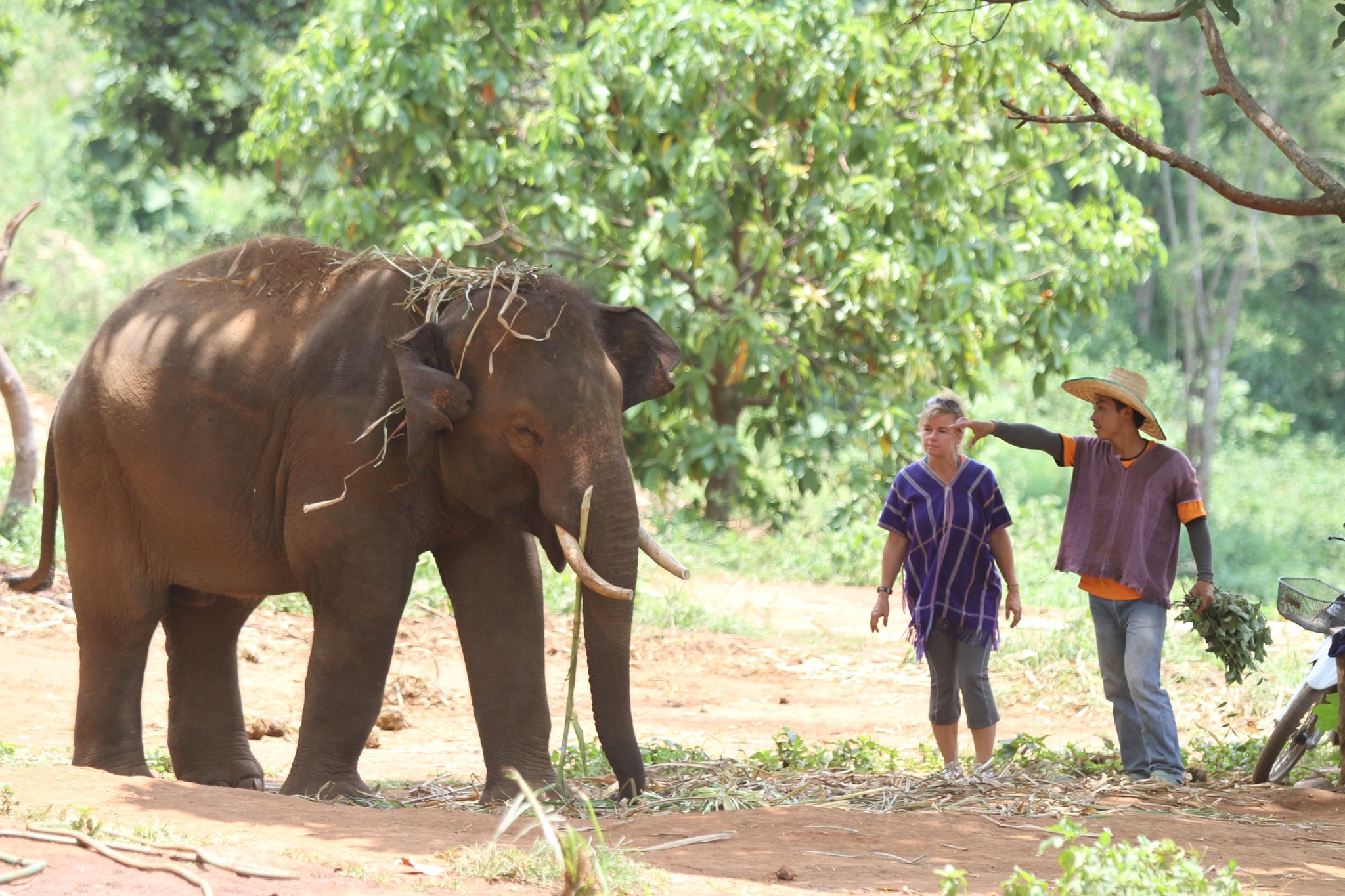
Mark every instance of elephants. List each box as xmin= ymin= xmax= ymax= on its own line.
xmin=0 ymin=236 xmax=692 ymax=809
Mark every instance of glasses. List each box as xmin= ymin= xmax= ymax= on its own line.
xmin=925 ymin=396 xmax=960 ymax=416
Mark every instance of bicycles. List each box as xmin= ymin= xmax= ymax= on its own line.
xmin=1250 ymin=522 xmax=1345 ymax=786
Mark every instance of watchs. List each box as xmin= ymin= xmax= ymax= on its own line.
xmin=877 ymin=586 xmax=892 ymax=594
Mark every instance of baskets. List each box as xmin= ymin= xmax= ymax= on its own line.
xmin=1277 ymin=577 xmax=1345 ymax=634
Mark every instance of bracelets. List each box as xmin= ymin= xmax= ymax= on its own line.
xmin=1007 ymin=583 xmax=1020 ymax=588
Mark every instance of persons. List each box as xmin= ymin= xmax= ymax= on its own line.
xmin=949 ymin=368 xmax=1215 ymax=790
xmin=871 ymin=400 xmax=1022 ymax=785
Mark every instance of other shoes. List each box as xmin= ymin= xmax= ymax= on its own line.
xmin=972 ymin=763 xmax=993 ymax=781
xmin=1127 ymin=772 xmax=1147 ymax=784
xmin=1147 ymin=768 xmax=1178 ymax=790
xmin=942 ymin=761 xmax=964 ymax=781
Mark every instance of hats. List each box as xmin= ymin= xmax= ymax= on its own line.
xmin=1061 ymin=366 xmax=1168 ymax=441
